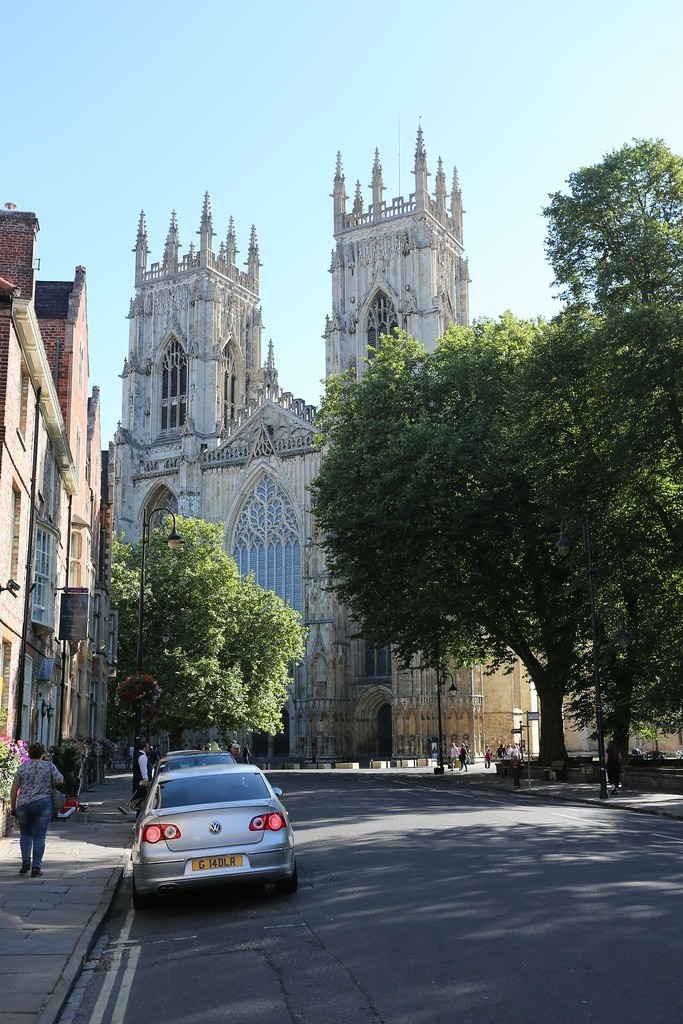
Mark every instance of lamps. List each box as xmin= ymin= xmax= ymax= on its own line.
xmin=37 ymin=699 xmax=45 ymax=707
xmin=42 ymin=705 xmax=48 ymax=718
xmin=48 ymin=708 xmax=54 ymax=721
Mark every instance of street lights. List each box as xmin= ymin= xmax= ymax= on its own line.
xmin=133 ymin=505 xmax=188 ymax=771
xmin=433 ymin=662 xmax=459 ymax=772
xmin=558 ymin=510 xmax=632 ymax=796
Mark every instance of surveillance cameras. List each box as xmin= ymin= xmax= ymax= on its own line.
xmin=8 ymin=579 xmax=21 ymax=591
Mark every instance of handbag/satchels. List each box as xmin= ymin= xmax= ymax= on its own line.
xmin=49 ymin=761 xmax=65 ymax=811
xmin=455 ymin=760 xmax=461 ymax=768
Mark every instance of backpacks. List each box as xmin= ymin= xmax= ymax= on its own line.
xmin=498 ymin=749 xmax=504 ymax=757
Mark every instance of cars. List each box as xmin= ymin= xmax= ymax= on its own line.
xmin=149 ymin=748 xmax=246 ymax=794
xmin=127 ymin=763 xmax=299 ymax=910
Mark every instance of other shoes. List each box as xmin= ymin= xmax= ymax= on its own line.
xmin=610 ymin=789 xmax=616 ymax=795
xmin=19 ymin=861 xmax=30 ymax=873
xmin=31 ymin=867 xmax=43 ymax=877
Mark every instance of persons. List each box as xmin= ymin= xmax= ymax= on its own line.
xmin=449 ymin=742 xmax=471 ymax=772
xmin=604 ymin=741 xmax=621 ymax=795
xmin=497 ymin=743 xmax=522 ymax=760
xmin=123 ymin=743 xmax=159 ymax=772
xmin=192 ymin=742 xmax=242 ymax=763
xmin=132 ymin=740 xmax=152 ymax=818
xmin=243 ymin=743 xmax=250 ymax=764
xmin=485 ymin=745 xmax=492 ymax=768
xmin=432 ymin=748 xmax=435 ymax=753
xmin=10 ymin=742 xmax=64 ymax=877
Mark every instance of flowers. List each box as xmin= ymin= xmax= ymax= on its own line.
xmin=63 ymin=732 xmax=118 ymax=768
xmin=114 ymin=671 xmax=162 ymax=719
xmin=0 ymin=735 xmax=31 ymax=813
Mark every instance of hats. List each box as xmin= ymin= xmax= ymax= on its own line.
xmin=233 ymin=744 xmax=241 ymax=749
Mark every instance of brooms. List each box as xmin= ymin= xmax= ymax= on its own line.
xmin=116 ymin=789 xmax=138 ymax=816
